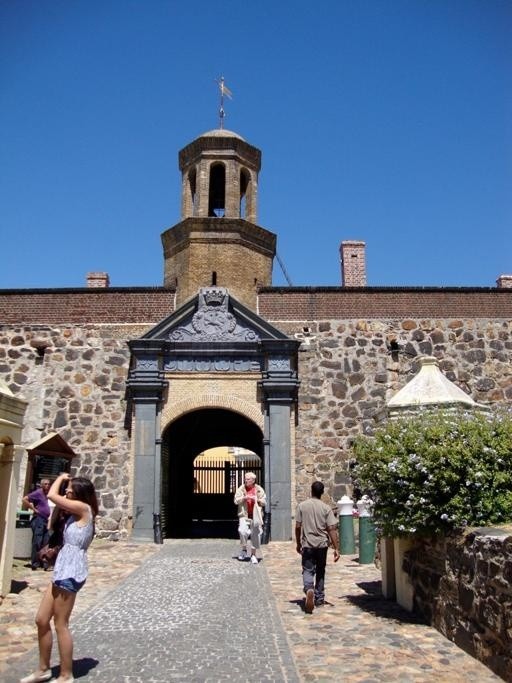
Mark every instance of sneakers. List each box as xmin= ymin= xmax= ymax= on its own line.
xmin=21 ymin=669 xmax=52 ymax=683
xmin=239 ymin=550 xmax=259 ymax=564
xmin=305 ymin=589 xmax=315 ymax=614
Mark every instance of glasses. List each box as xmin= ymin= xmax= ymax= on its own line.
xmin=65 ymin=489 xmax=74 ymax=495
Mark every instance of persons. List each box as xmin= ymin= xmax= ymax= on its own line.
xmin=20 ymin=477 xmax=52 ymax=571
xmin=20 ymin=472 xmax=100 ymax=683
xmin=231 ymin=470 xmax=268 ymax=564
xmin=294 ymin=480 xmax=340 ymax=613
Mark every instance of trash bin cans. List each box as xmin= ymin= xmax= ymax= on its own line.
xmin=13 ymin=519 xmax=33 ymax=558
